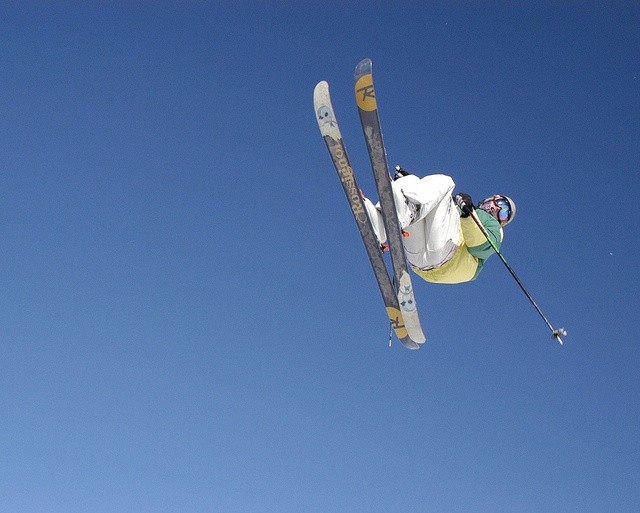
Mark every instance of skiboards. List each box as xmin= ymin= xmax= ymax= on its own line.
xmin=313 ymin=58 xmax=427 ymax=350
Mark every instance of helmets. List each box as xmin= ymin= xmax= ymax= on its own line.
xmin=479 ymin=195 xmax=516 ymax=227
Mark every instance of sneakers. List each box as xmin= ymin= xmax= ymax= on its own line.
xmin=391 ymin=180 xmax=413 ymax=230
xmin=362 ymin=198 xmax=387 ymax=244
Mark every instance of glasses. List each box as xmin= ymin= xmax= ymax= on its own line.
xmin=493 ymin=195 xmax=511 ymax=225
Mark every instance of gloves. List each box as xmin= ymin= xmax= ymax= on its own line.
xmin=393 ymin=168 xmax=410 ymax=181
xmin=453 ymin=193 xmax=476 ymax=217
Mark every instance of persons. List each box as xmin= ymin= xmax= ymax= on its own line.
xmin=359 ymin=173 xmax=516 ymax=285
